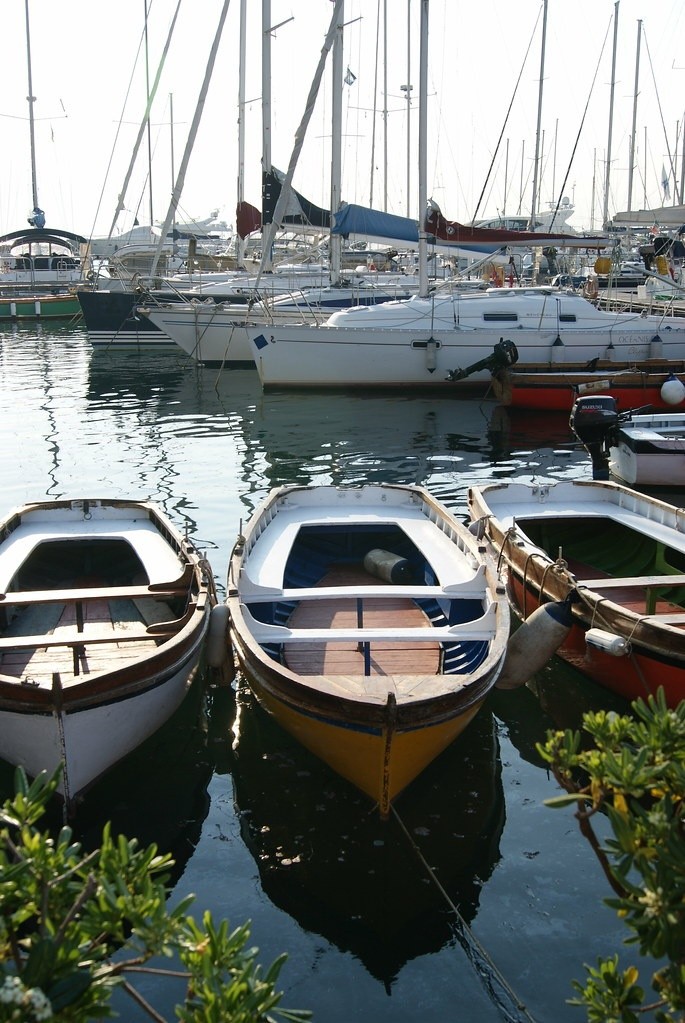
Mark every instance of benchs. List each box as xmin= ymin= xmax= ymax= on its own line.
xmin=579 ymin=574 xmax=685 ymax=590
xmin=0 ymin=624 xmax=183 ymax=651
xmin=253 ymin=624 xmax=493 ymax=646
xmin=642 ymin=613 xmax=685 ymax=625
xmin=242 ymin=585 xmax=488 ymax=603
xmin=0 ymin=584 xmax=194 ymax=599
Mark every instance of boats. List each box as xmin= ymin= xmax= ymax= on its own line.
xmin=0 ymin=500 xmax=215 ymax=809
xmin=469 ymin=476 xmax=685 ymax=722
xmin=221 ymin=484 xmax=511 ymax=820
xmin=490 ymin=359 xmax=685 ymax=412
xmin=605 ymin=413 xmax=685 ymax=491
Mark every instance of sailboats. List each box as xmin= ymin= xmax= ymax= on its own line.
xmin=0 ymin=0 xmax=91 ymax=320
xmin=77 ymin=0 xmax=685 ymax=392
